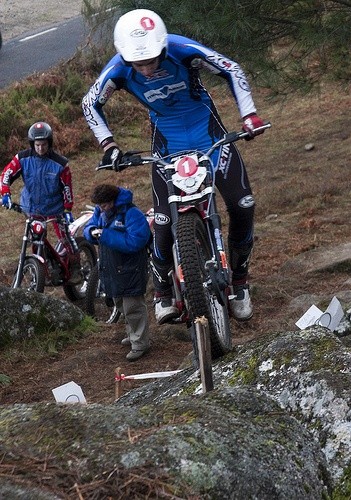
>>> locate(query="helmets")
[113,9,168,66]
[28,122,53,149]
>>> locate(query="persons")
[0,122,90,293]
[83,9,263,322]
[83,184,152,360]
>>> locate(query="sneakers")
[227,280,254,321]
[152,297,179,325]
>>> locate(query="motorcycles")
[80,204,158,325]
[95,123,275,361]
[1,199,97,302]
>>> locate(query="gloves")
[2,195,12,209]
[241,116,264,140]
[63,212,73,224]
[102,146,123,173]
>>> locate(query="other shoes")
[126,350,144,359]
[121,337,131,346]
[68,270,82,282]
